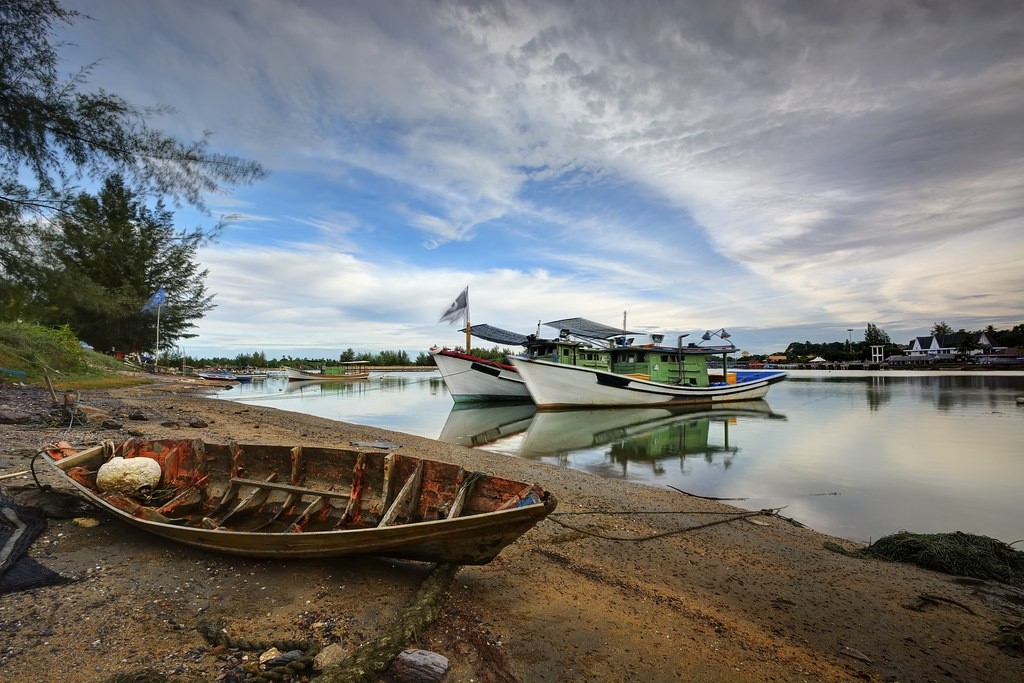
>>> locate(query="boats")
[503,308,792,412]
[515,397,788,477]
[195,371,270,381]
[37,435,559,567]
[281,365,370,381]
[439,400,540,448]
[428,324,611,404]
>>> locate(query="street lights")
[846,329,853,352]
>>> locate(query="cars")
[763,365,774,369]
[79,341,95,350]
[136,354,151,364]
